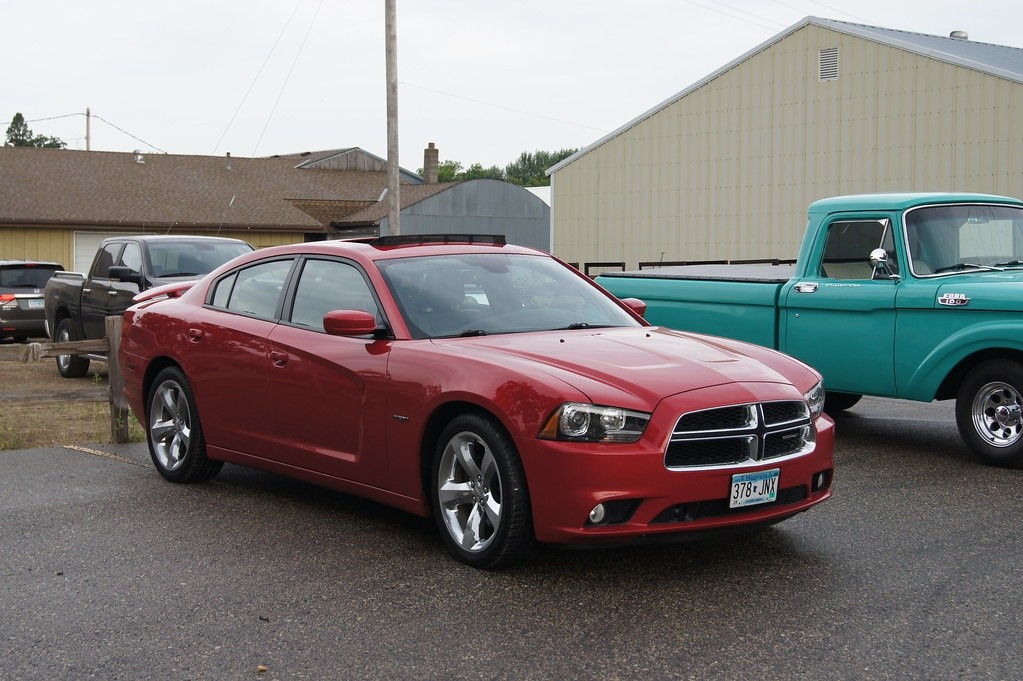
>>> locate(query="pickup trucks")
[590,192,1023,470]
[42,234,259,379]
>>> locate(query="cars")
[0,259,66,344]
[118,230,834,570]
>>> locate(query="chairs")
[410,266,485,340]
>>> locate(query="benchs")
[817,260,934,281]
